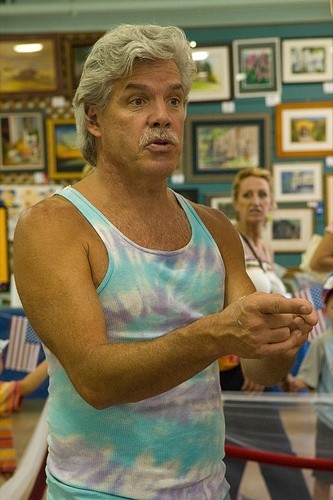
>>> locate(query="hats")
[321,273,333,304]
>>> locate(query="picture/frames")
[276,101,333,158]
[231,36,282,99]
[184,114,269,183]
[0,31,106,180]
[188,43,233,105]
[282,36,333,85]
[204,162,333,254]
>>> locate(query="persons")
[13,24,318,500]
[223,168,312,500]
[308,222,333,273]
[0,342,47,482]
[278,289,333,500]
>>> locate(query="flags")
[6,316,40,374]
[300,285,325,340]
